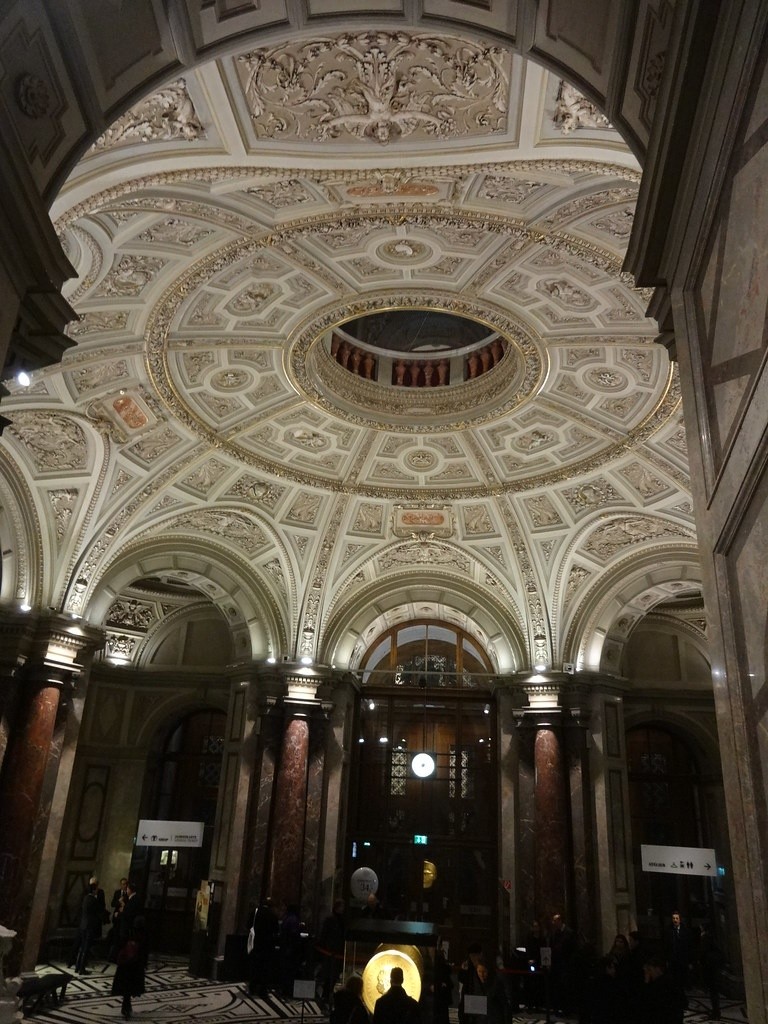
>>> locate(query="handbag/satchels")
[114,928,139,968]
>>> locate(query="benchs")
[17,973,73,1018]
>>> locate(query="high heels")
[121,1000,133,1021]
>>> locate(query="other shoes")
[75,969,92,975]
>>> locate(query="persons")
[332,975,370,1024]
[455,945,512,1024]
[110,903,151,1022]
[67,876,111,975]
[106,878,141,965]
[373,968,423,1024]
[665,912,722,1021]
[525,914,581,1014]
[425,944,454,1024]
[588,930,687,1024]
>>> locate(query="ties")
[675,925,678,936]
[122,891,125,896]
